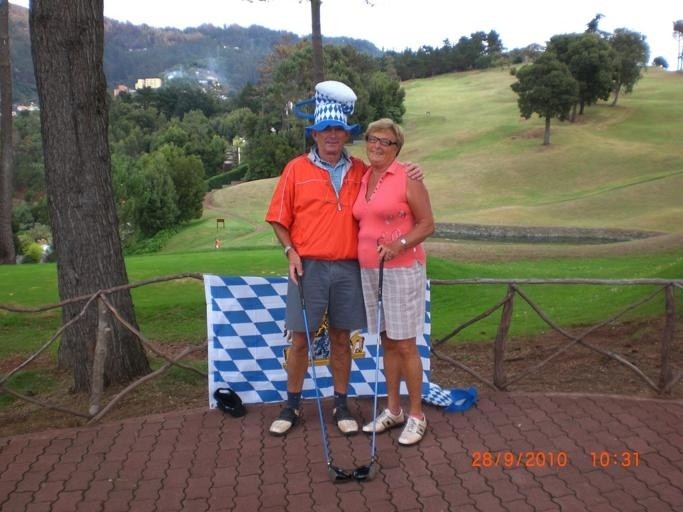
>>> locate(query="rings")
[388,255,392,259]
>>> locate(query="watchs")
[396,236,410,252]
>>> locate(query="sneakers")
[362,408,404,432]
[269,406,303,436]
[398,414,427,445]
[333,405,359,435]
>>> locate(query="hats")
[213,387,246,416]
[304,103,360,139]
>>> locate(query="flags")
[201,272,432,411]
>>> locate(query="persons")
[265,80,424,437]
[349,118,436,446]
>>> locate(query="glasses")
[365,134,398,146]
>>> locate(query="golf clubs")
[297,258,351,483]
[352,254,383,481]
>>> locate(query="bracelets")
[283,245,294,256]
